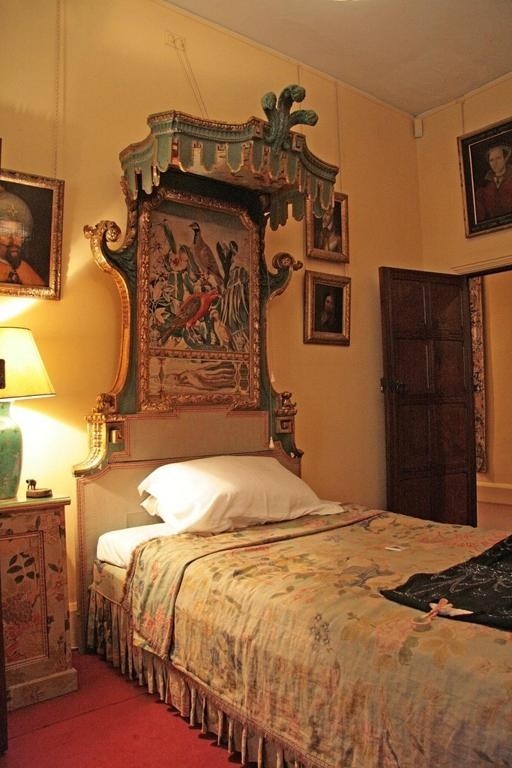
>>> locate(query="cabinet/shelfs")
[1,168,65,302]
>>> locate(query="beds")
[73,82,512,768]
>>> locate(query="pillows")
[136,455,345,536]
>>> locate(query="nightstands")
[0,496,79,713]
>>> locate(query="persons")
[0,191,47,286]
[475,144,512,222]
[315,209,343,253]
[315,294,341,331]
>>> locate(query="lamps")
[0,326,57,499]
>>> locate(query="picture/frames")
[456,117,512,239]
[304,190,349,264]
[303,268,352,347]
